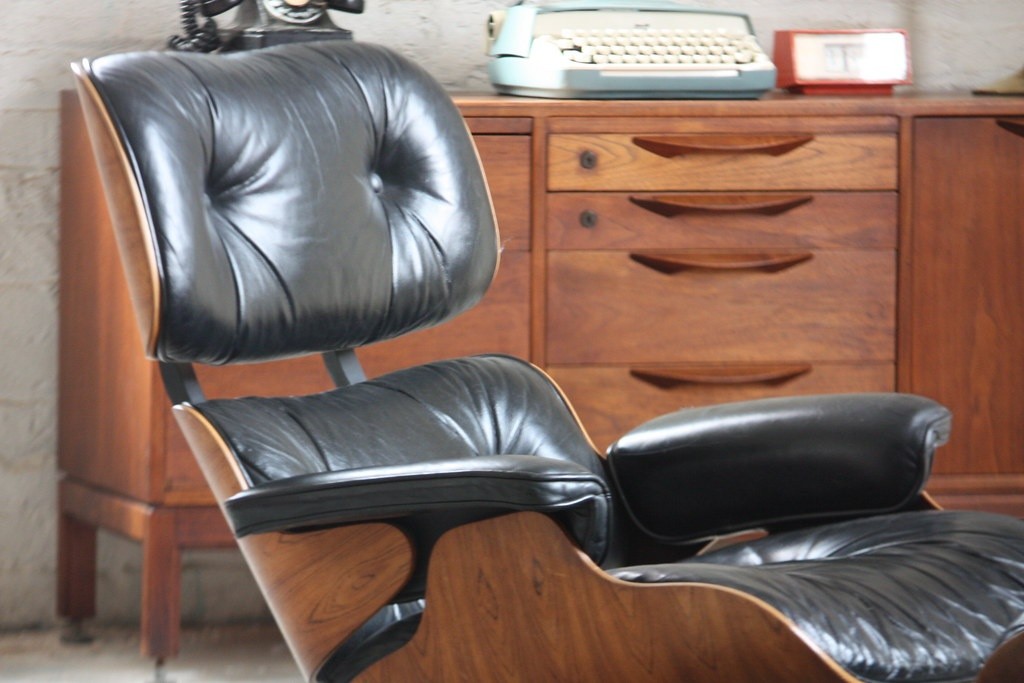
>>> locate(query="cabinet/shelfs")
[53,84,535,658]
[898,98,1023,523]
[537,100,899,456]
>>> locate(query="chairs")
[70,40,1024,682]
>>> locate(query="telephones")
[166,0,365,57]
